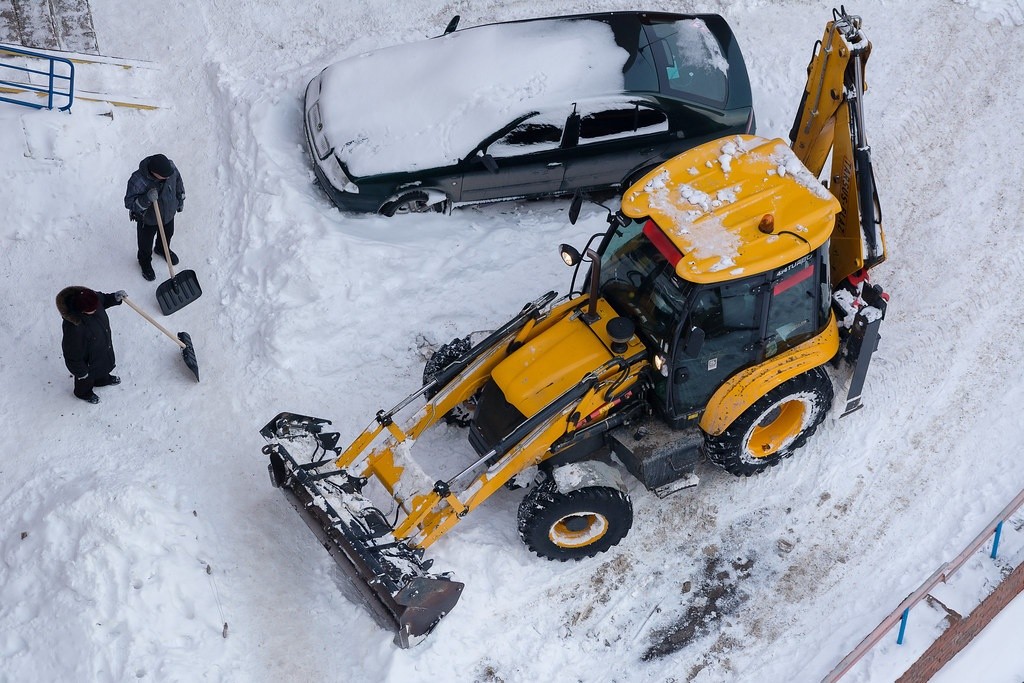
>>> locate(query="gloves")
[78,373,88,380]
[148,188,158,201]
[115,290,128,301]
[177,205,184,212]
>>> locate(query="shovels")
[122,296,199,382]
[154,199,202,316]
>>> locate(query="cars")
[303,12,759,215]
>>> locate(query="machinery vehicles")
[258,9,891,647]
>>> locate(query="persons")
[124,153,186,282]
[55,286,129,404]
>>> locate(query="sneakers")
[138,260,156,281]
[154,247,179,265]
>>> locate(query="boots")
[90,372,120,387]
[73,377,100,404]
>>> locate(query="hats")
[77,290,99,312]
[149,154,174,177]
